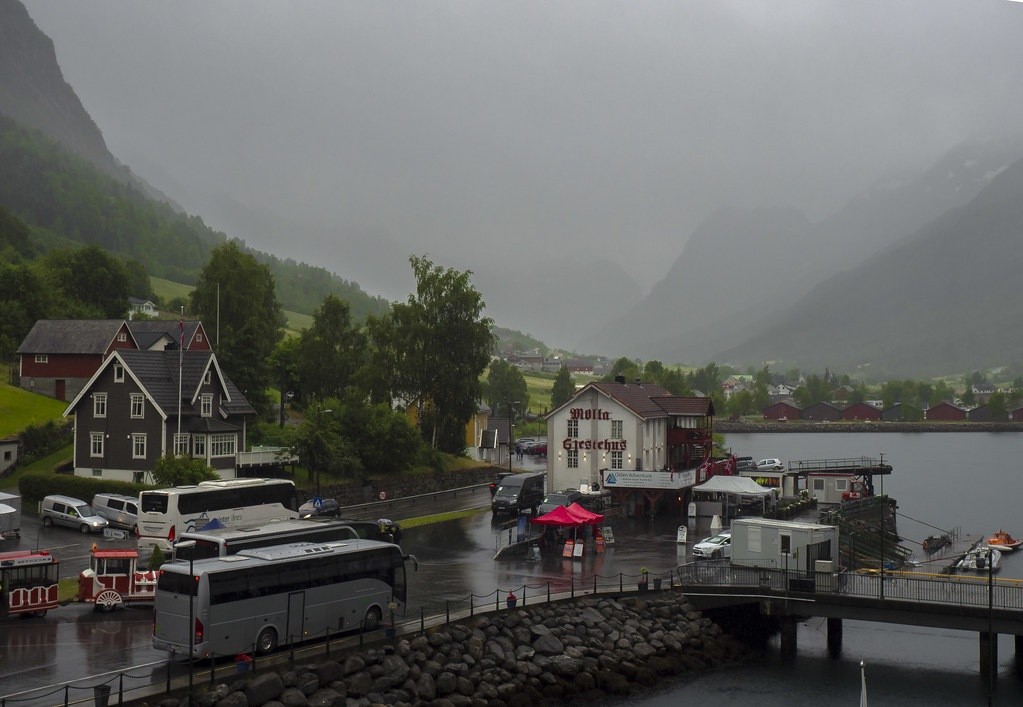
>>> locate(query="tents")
[529,504,592,543]
[691,475,778,516]
[568,502,604,527]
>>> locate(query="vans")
[37,495,109,534]
[92,493,139,536]
[491,474,545,517]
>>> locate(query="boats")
[956,527,1023,573]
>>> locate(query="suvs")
[735,456,784,472]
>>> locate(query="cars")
[693,533,731,562]
[299,499,342,519]
[539,489,583,516]
[514,438,547,457]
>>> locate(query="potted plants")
[765,490,818,519]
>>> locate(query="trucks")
[0,492,22,540]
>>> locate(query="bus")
[0,543,160,620]
[138,478,301,552]
[151,518,419,660]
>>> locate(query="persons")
[515,443,524,460]
[886,560,895,577]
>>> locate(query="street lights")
[509,401,520,472]
[315,406,333,427]
[878,452,887,599]
[537,401,541,441]
